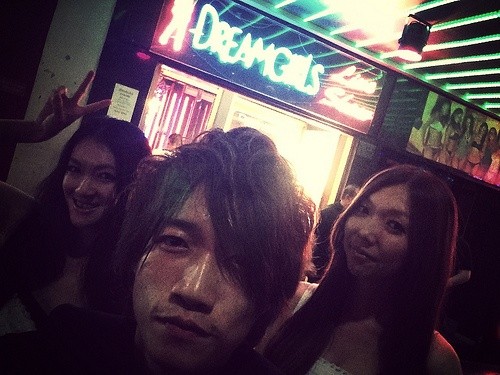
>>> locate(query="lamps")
[396,13,432,63]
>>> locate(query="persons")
[419,97,500,186]
[0,71,463,375]
